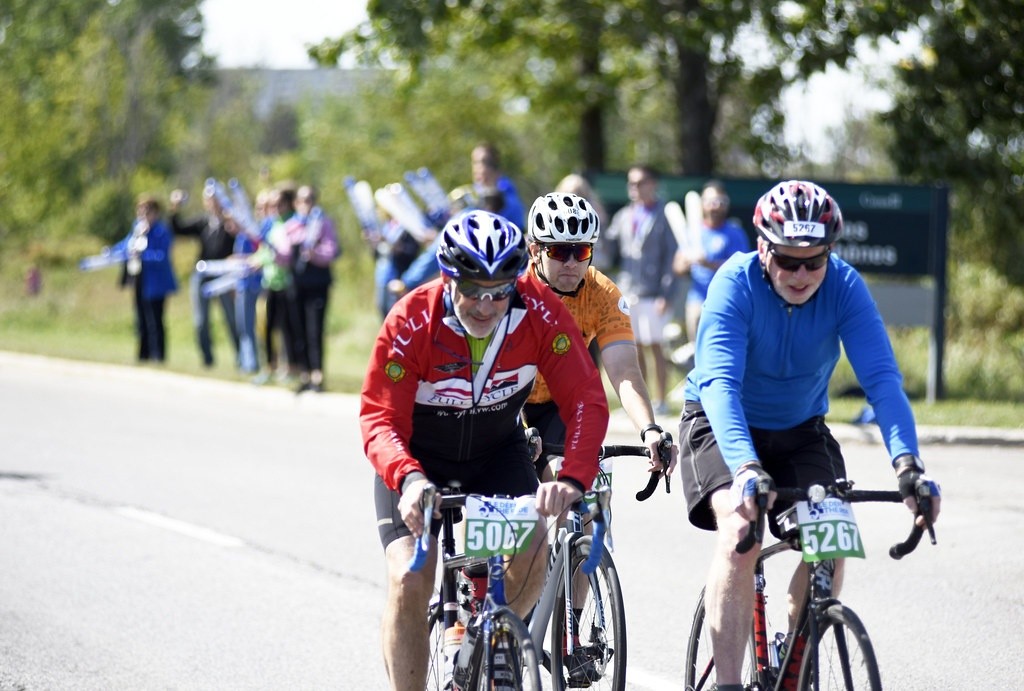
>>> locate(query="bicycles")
[525,425,671,691]
[407,480,615,691]
[684,475,937,691]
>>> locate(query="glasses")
[766,243,833,273]
[451,277,516,301]
[536,242,595,262]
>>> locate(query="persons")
[369,140,526,320]
[556,173,612,276]
[166,185,338,393]
[363,209,609,691]
[114,197,178,362]
[608,164,678,413]
[443,192,679,691]
[678,178,941,691]
[672,183,749,360]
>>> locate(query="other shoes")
[561,646,596,688]
[777,643,789,670]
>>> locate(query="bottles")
[457,578,478,626]
[442,615,485,691]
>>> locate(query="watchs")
[641,424,664,442]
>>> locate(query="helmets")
[435,210,529,280]
[528,192,601,244]
[752,180,845,249]
